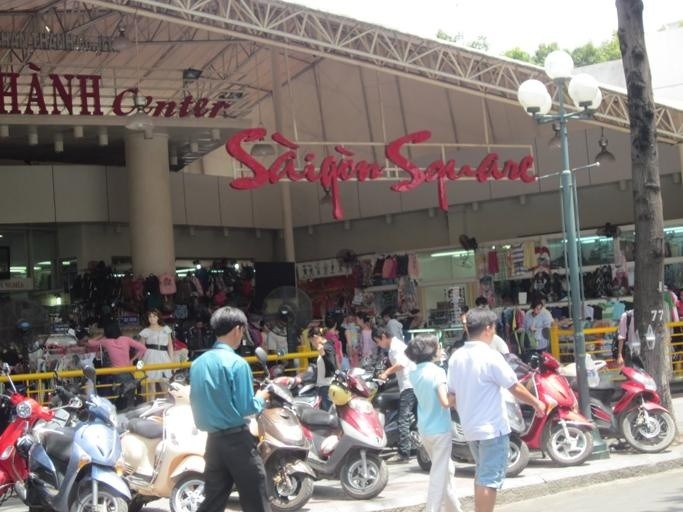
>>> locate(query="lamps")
[593,127,616,163]
[548,121,562,149]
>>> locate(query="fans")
[596,223,621,238]
[0,299,51,375]
[262,285,313,370]
[336,247,357,268]
[459,234,478,250]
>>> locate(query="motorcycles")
[366,347,677,479]
[0,348,390,512]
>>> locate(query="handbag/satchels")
[611,338,634,364]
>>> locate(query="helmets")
[15,432,33,457]
[329,380,355,406]
[320,435,338,459]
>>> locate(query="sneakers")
[385,454,410,464]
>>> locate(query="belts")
[211,425,249,440]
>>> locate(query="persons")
[188,306,272,512]
[403,334,465,512]
[446,306,547,512]
[80,319,147,397]
[137,307,174,404]
[370,326,420,466]
[663,285,683,372]
[249,306,404,412]
[223,264,255,312]
[475,295,554,364]
[459,312,509,361]
[617,309,644,376]
